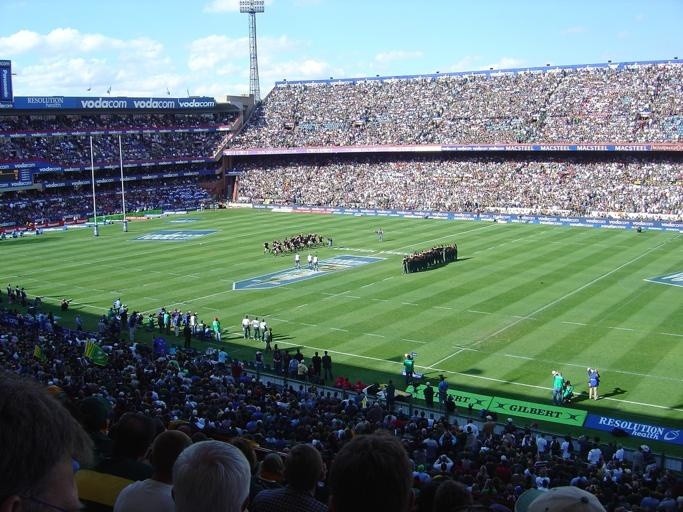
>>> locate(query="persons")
[0,64,683,511]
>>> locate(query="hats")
[515,486,606,512]
